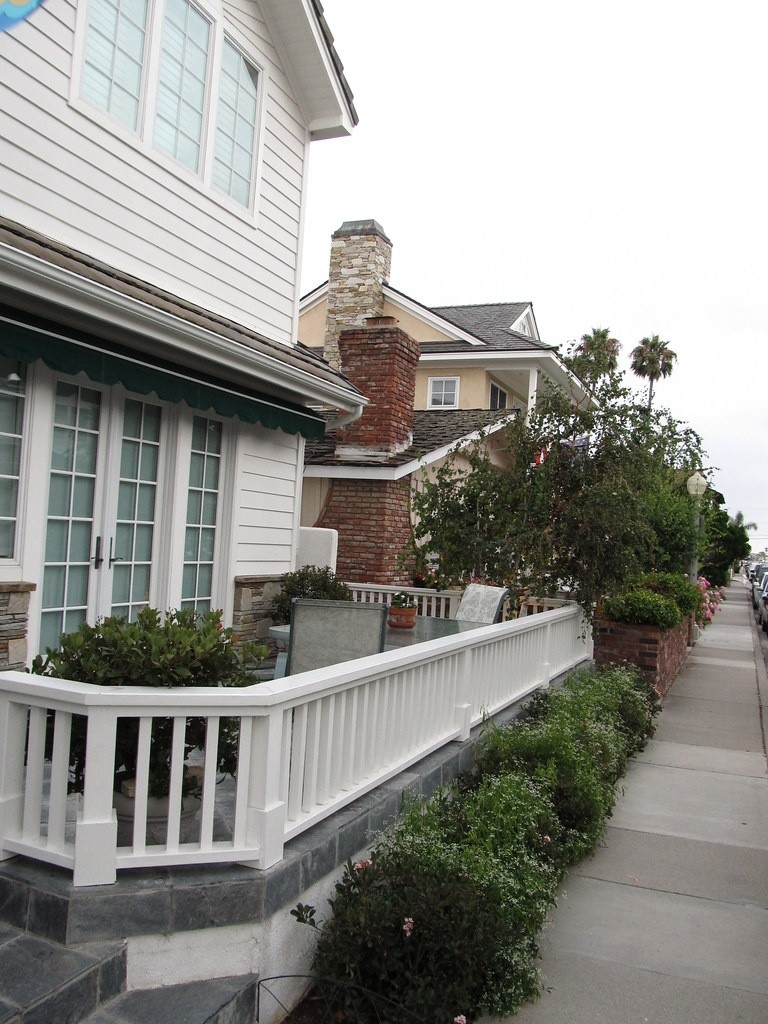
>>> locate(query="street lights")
[686,471,707,647]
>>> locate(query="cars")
[741,558,768,637]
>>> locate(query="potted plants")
[20,604,270,848]
[269,564,354,652]
[387,590,418,629]
